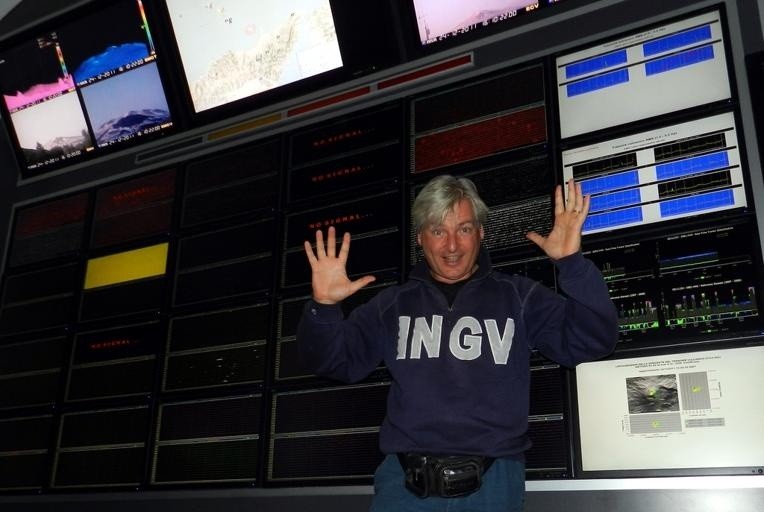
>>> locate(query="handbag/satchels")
[398,452,492,499]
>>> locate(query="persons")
[294,174,620,512]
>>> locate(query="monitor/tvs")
[580,215,763,352]
[550,3,739,146]
[552,103,756,246]
[566,338,764,479]
